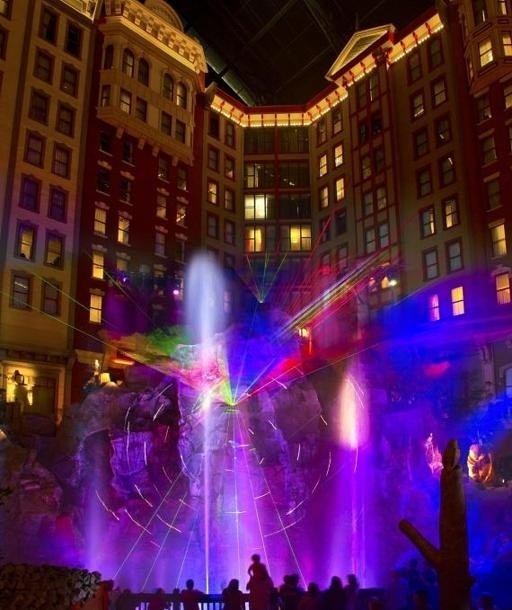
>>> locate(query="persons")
[2,554,495,609]
